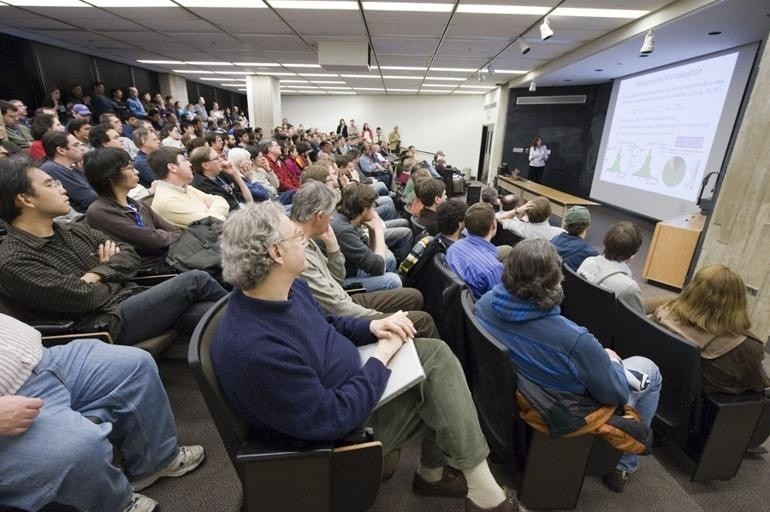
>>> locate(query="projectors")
[318,41,373,74]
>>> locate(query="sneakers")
[123,493,160,512]
[465,489,530,512]
[132,445,205,492]
[601,468,636,492]
[414,464,468,497]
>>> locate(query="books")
[356,335,426,415]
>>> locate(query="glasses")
[282,231,304,241]
[48,180,62,193]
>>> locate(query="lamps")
[528,78,539,92]
[517,17,558,57]
[638,31,658,54]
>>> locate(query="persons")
[646,265,770,460]
[211,198,523,512]
[0,82,657,348]
[0,310,205,512]
[471,238,664,492]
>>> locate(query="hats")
[73,103,92,115]
[564,206,591,226]
